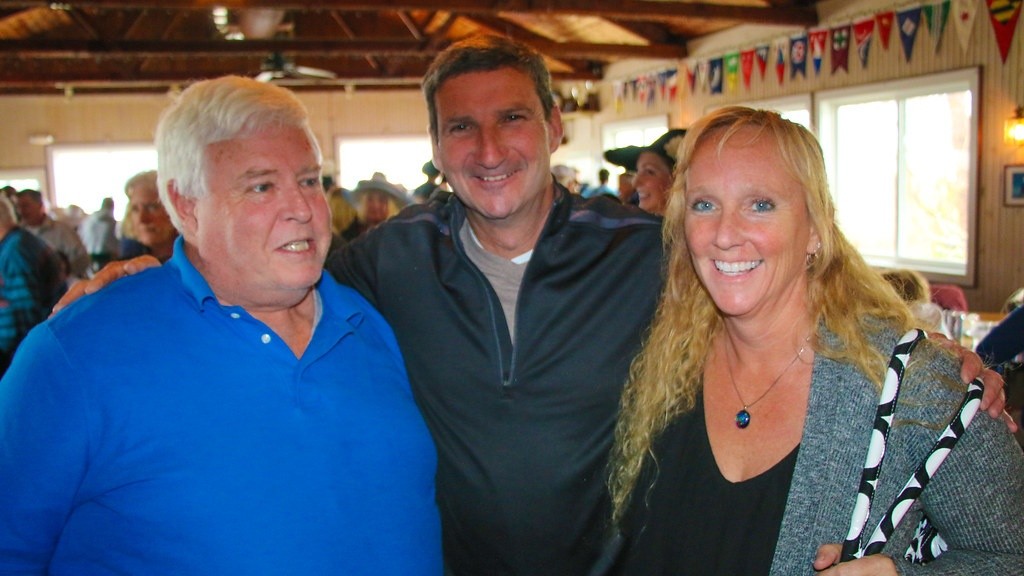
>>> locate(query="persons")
[47,33,1017,576]
[600,107,1024,576]
[0,75,443,575]
[882,269,1024,370]
[0,129,692,379]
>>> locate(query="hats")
[603,129,687,170]
[357,172,405,206]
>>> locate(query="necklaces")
[723,331,810,430]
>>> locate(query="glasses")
[127,201,164,215]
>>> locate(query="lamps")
[1004,104,1024,145]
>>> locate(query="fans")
[254,52,336,82]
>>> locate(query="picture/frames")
[1003,164,1024,207]
